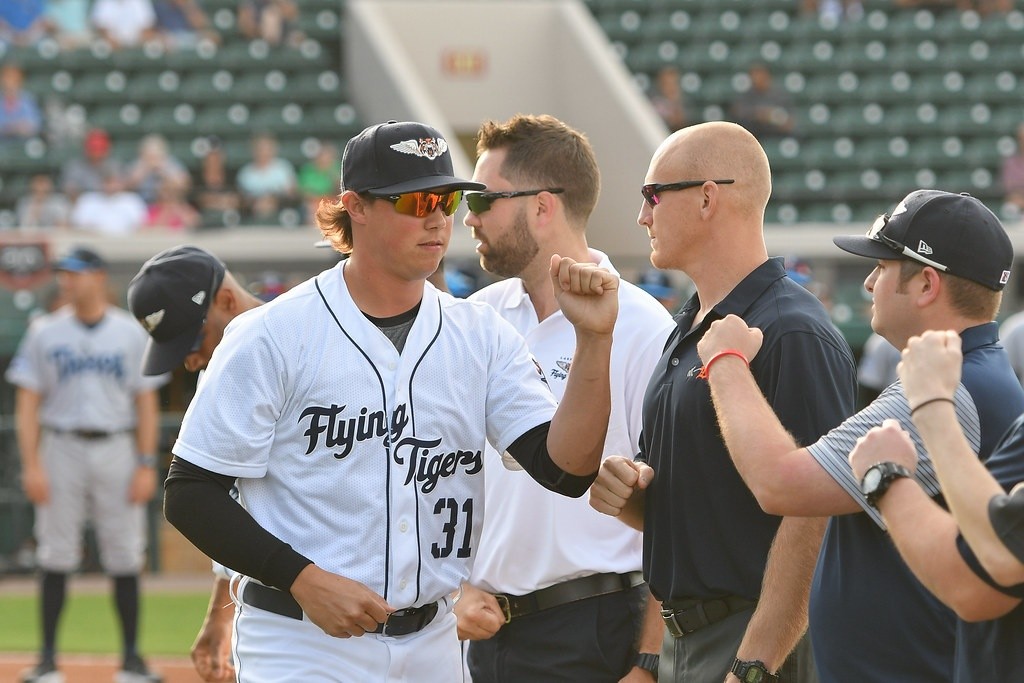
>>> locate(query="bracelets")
[139,455,159,465]
[909,398,956,416]
[634,652,659,683]
[704,349,749,379]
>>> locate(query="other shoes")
[116,663,160,683]
[23,665,57,683]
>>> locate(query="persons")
[697,189,1024,683]
[17,128,342,233]
[0,63,42,138]
[3,250,171,683]
[652,62,796,134]
[463,115,678,683]
[0,0,308,51]
[164,121,619,683]
[637,121,859,683]
[634,269,677,312]
[896,330,1024,587]
[128,244,265,683]
[848,413,1024,683]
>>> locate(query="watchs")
[860,462,912,511]
[731,657,779,683]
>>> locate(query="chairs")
[581,0,1024,232]
[0,0,373,230]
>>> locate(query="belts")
[494,567,645,624]
[660,586,767,640]
[76,429,107,439]
[244,583,448,638]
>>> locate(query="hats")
[342,119,487,196]
[86,129,112,157]
[125,245,227,376]
[55,247,106,272]
[833,188,1014,290]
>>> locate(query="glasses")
[865,212,948,272]
[465,187,567,217]
[361,188,463,218]
[641,178,735,208]
